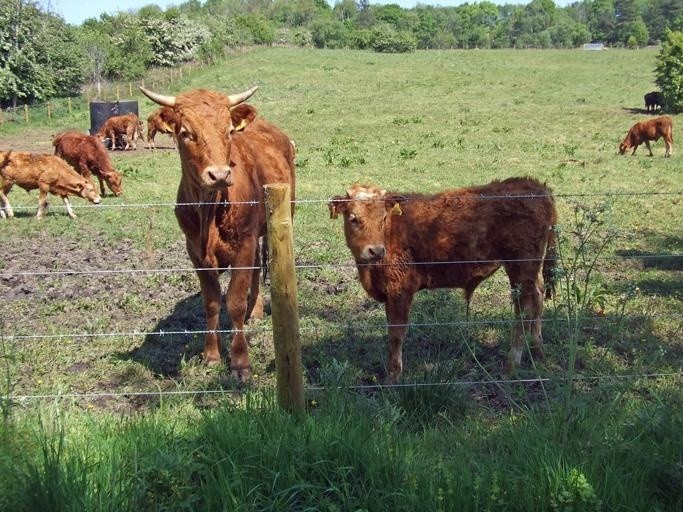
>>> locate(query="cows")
[329,177,558,385]
[619,117,673,158]
[147,109,173,152]
[95,113,145,151]
[137,85,297,384]
[0,151,103,221]
[644,91,661,112]
[53,131,123,198]
